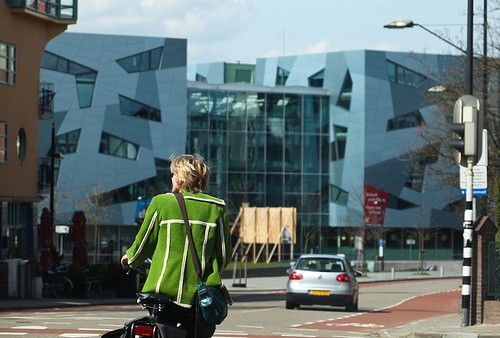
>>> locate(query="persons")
[121,154,233,338]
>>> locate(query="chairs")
[309,263,316,270]
[332,264,341,271]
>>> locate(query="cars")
[286,253,359,312]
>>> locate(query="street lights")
[384,17,486,131]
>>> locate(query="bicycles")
[121,256,172,338]
[42,268,73,298]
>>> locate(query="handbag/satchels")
[195,281,233,325]
